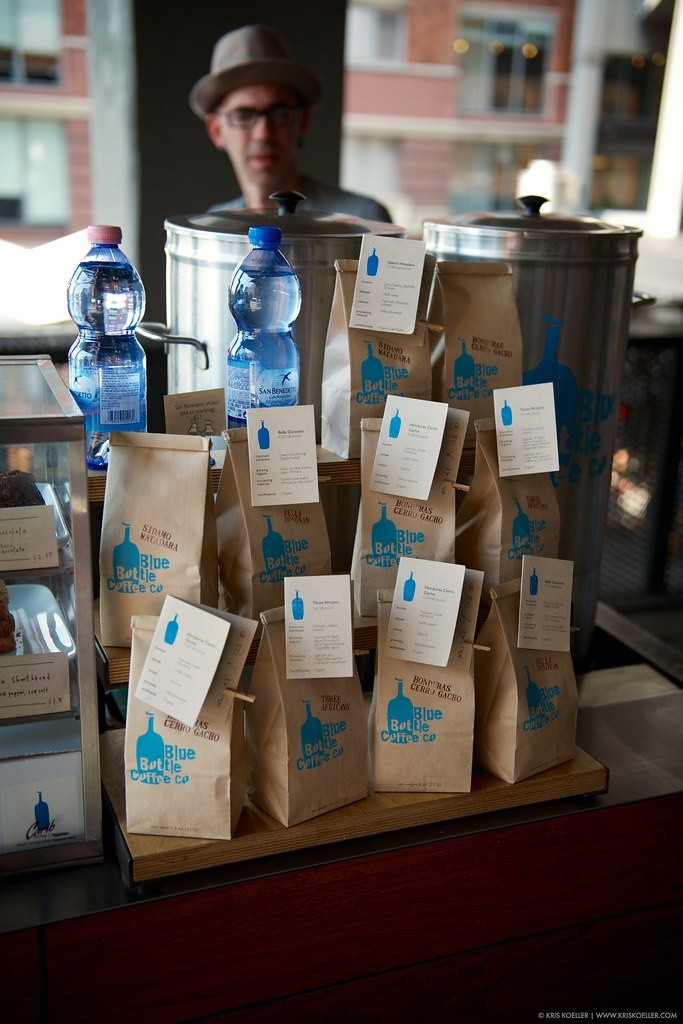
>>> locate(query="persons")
[189,25,391,223]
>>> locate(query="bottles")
[67,223,149,470]
[227,227,302,427]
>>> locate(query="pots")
[164,190,410,398]
[422,196,657,664]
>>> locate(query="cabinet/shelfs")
[0,353,104,880]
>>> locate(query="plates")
[7,583,77,662]
[35,482,70,550]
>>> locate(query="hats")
[190,25,324,121]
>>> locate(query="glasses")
[217,105,302,127]
